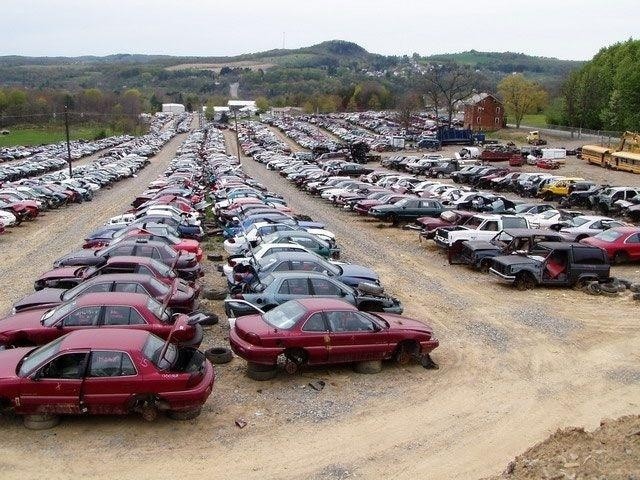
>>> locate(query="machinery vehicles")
[526,131,547,146]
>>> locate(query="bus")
[580,146,610,167]
[610,152,640,172]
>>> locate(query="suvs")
[489,242,610,290]
[433,215,531,251]
[461,228,565,270]
[450,164,586,200]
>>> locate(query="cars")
[0,164,90,234]
[227,233,381,290]
[262,153,448,223]
[528,209,585,232]
[0,278,203,350]
[230,109,464,159]
[34,251,200,313]
[580,226,640,265]
[132,171,287,207]
[246,224,332,260]
[0,314,214,415]
[89,165,131,199]
[107,206,204,239]
[223,217,340,256]
[83,227,203,262]
[12,274,200,314]
[134,135,240,170]
[143,110,228,134]
[557,207,634,242]
[223,240,345,290]
[84,215,200,242]
[0,135,132,164]
[223,264,402,319]
[229,298,438,367]
[53,238,204,281]
[222,214,325,238]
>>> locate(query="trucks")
[430,161,471,179]
[527,148,566,166]
